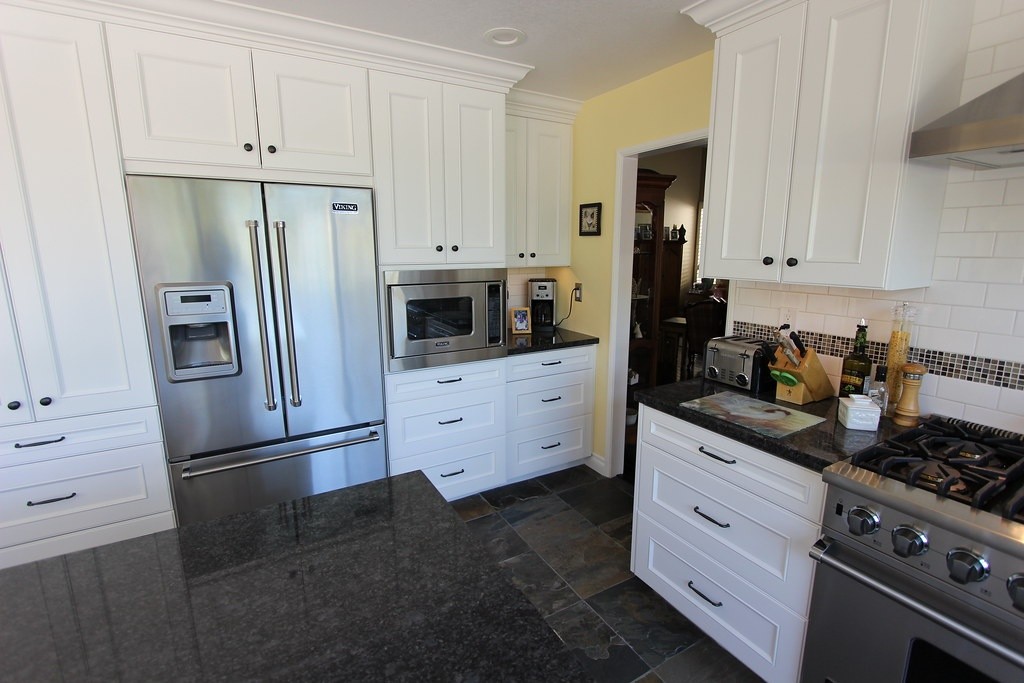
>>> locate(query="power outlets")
[779,306,799,335]
[575,282,582,302]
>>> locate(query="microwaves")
[381,266,508,374]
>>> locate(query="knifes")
[762,327,807,369]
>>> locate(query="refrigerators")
[127,173,388,529]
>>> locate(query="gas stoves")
[821,415,1023,626]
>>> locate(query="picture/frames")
[579,202,602,237]
[510,307,532,334]
[638,224,651,234]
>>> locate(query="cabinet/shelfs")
[629,168,687,385]
[0,0,599,570]
[680,0,973,291]
[629,403,828,683]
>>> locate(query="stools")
[661,317,687,368]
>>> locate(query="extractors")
[908,72,1024,169]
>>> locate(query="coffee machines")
[527,279,557,330]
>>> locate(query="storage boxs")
[838,397,881,432]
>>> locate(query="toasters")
[705,334,780,393]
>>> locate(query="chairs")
[684,300,718,365]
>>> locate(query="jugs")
[533,303,552,325]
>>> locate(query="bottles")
[671,224,678,240]
[838,319,873,405]
[883,301,914,409]
[678,224,686,240]
[869,365,890,416]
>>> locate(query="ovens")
[798,533,1023,683]
